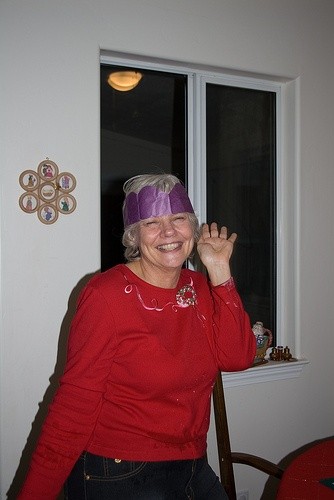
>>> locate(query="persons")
[18,175,256,500]
